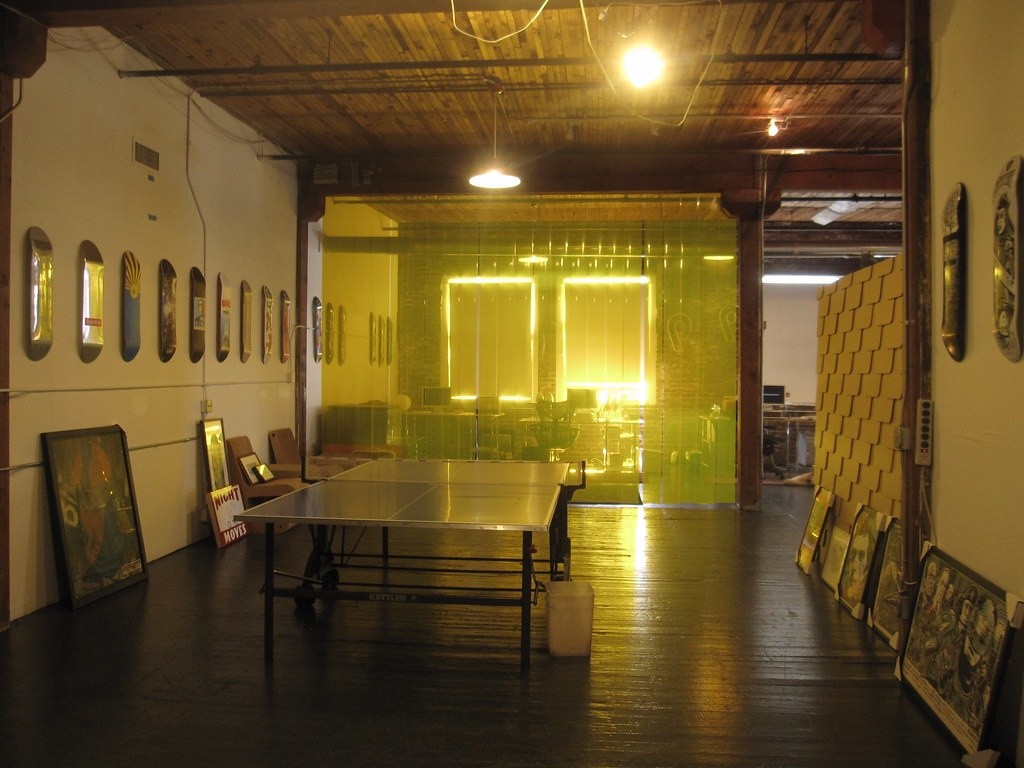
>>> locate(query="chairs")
[469,394,499,461]
[225,435,311,534]
[269,428,345,478]
[530,401,581,462]
[763,426,785,480]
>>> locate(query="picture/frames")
[833,501,887,620]
[200,416,231,492]
[795,485,837,576]
[252,463,276,483]
[865,514,930,651]
[892,540,1024,768]
[41,425,149,611]
[237,452,262,487]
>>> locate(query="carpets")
[568,484,643,505]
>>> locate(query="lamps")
[469,93,521,188]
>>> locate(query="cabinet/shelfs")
[697,413,733,471]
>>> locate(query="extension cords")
[915,399,933,464]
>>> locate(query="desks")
[517,417,645,474]
[267,463,322,481]
[232,481,562,669]
[405,410,506,460]
[315,456,570,595]
